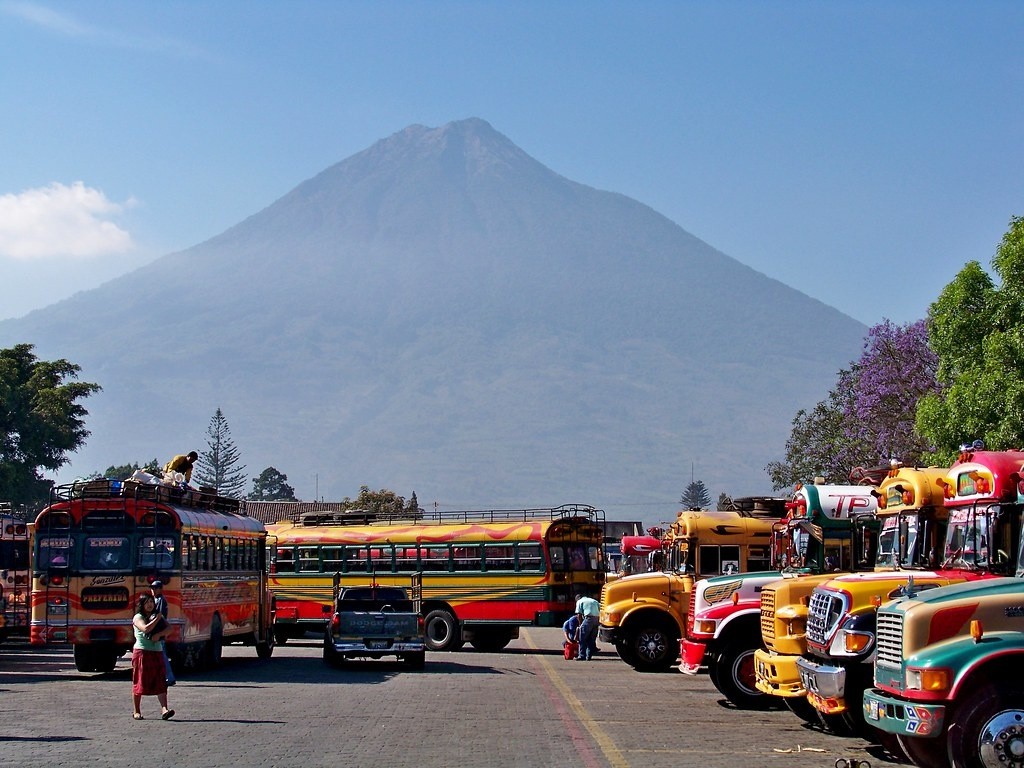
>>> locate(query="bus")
[257,502,608,655]
[34,477,274,674]
[0,503,37,639]
[598,439,1024,768]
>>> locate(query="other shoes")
[161,709,175,720]
[132,712,144,720]
[575,657,591,661]
[165,680,176,686]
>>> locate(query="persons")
[562,593,602,662]
[145,580,169,643]
[130,593,173,719]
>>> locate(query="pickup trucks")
[320,568,426,670]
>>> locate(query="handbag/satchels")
[143,614,167,641]
[565,642,579,661]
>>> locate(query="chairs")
[140,544,176,569]
[276,550,544,573]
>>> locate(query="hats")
[569,617,580,630]
[148,581,163,590]
